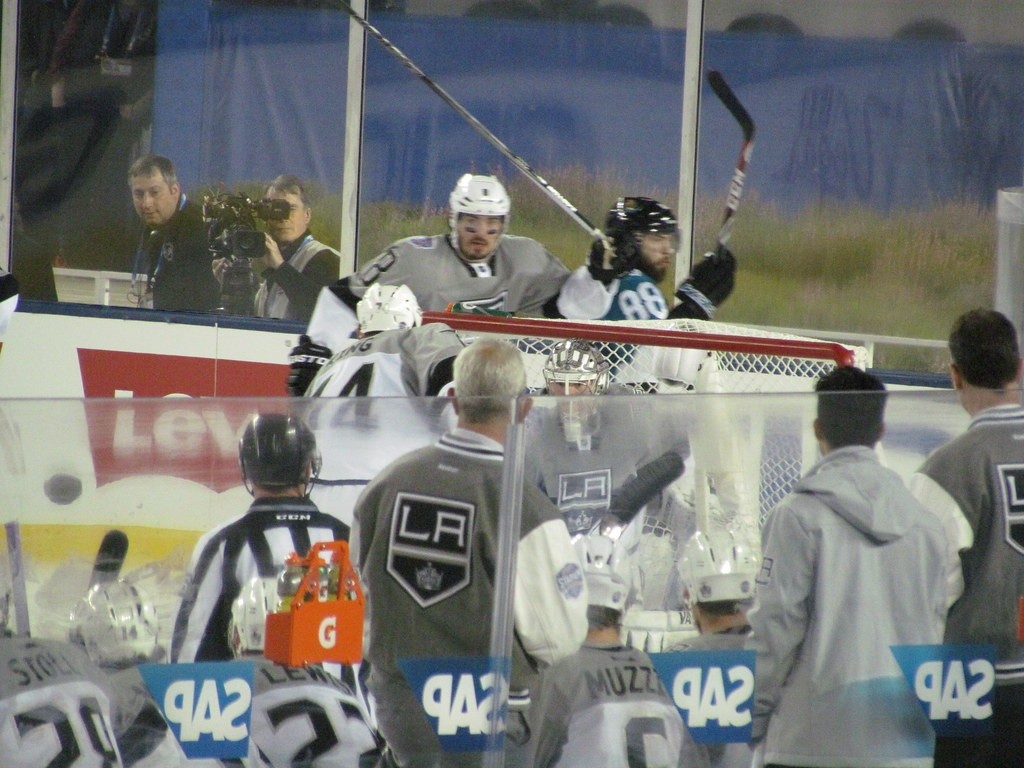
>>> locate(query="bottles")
[276,554,356,614]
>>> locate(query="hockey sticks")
[705,66,758,258]
[601,451,685,545]
[347,4,602,240]
[4,520,30,639]
[87,527,130,611]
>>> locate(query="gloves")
[672,244,737,321]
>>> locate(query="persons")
[130,155,220,311]
[212,180,339,323]
[287,174,639,424]
[15,1,153,303]
[521,195,754,768]
[350,339,589,768]
[917,311,1024,768]
[751,366,947,768]
[306,281,461,528]
[0,412,390,768]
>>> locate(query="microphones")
[219,206,235,226]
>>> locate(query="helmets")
[542,338,611,398]
[634,520,764,609]
[446,172,513,265]
[69,580,160,669]
[354,282,425,333]
[604,195,685,283]
[228,577,277,654]
[570,531,636,614]
[237,413,325,502]
